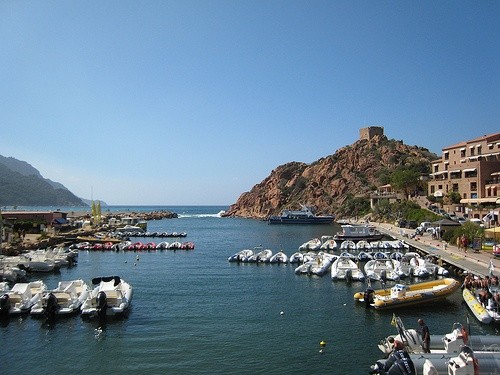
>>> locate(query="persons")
[493,292,500,314]
[388,337,404,352]
[432,228,437,239]
[461,234,465,250]
[447,207,450,213]
[415,318,431,353]
[478,290,492,308]
[462,238,468,253]
[463,272,499,289]
[456,236,461,251]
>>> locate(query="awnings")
[55,218,67,224]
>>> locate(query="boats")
[268,209,335,224]
[29,280,89,316]
[333,224,385,242]
[0,248,78,280]
[0,280,48,315]
[228,249,449,281]
[299,238,408,250]
[369,328,500,375]
[80,276,132,317]
[108,230,187,238]
[61,241,194,249]
[354,278,460,311]
[463,285,500,323]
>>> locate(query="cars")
[397,219,435,233]
[428,205,484,228]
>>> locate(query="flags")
[483,213,494,222]
